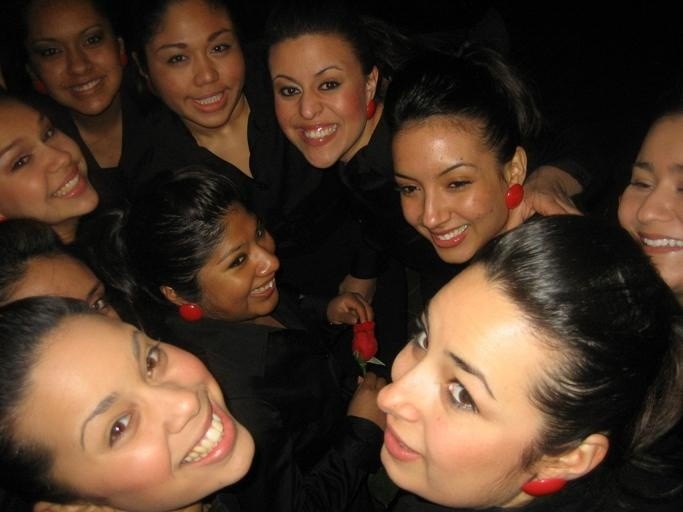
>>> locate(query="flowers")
[351,322,386,377]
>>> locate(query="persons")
[2,0,683,511]
